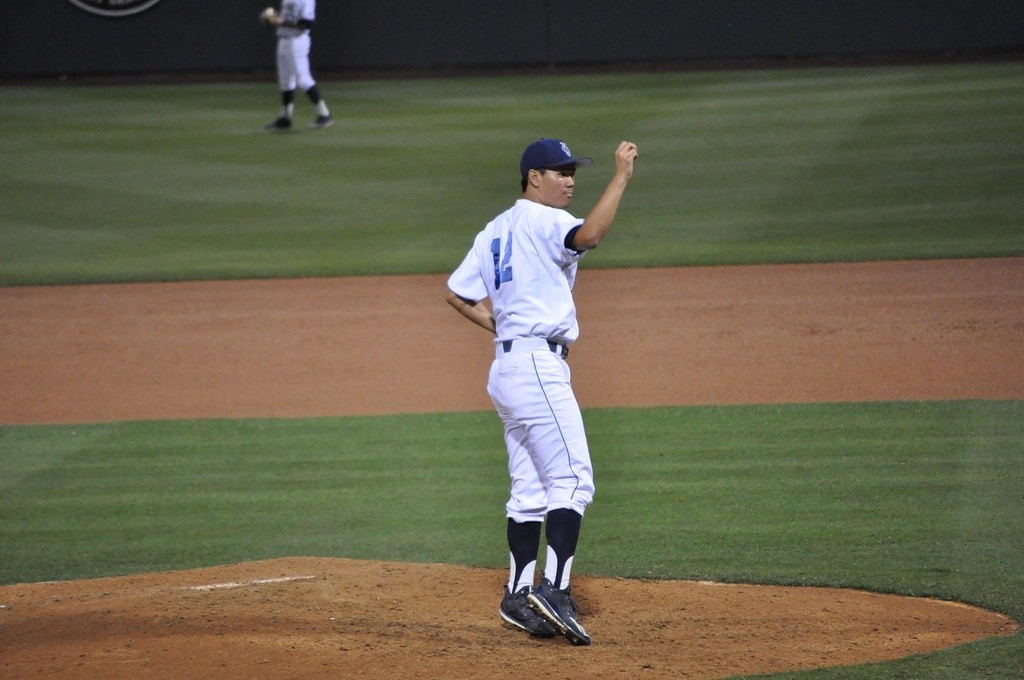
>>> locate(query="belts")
[502,339,569,358]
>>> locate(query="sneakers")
[500,585,557,636]
[526,577,591,645]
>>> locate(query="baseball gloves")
[259,6,280,28]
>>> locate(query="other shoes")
[308,115,334,128]
[264,117,292,130]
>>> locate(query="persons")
[259,0,335,132]
[446,139,638,647]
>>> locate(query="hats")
[519,138,593,173]
